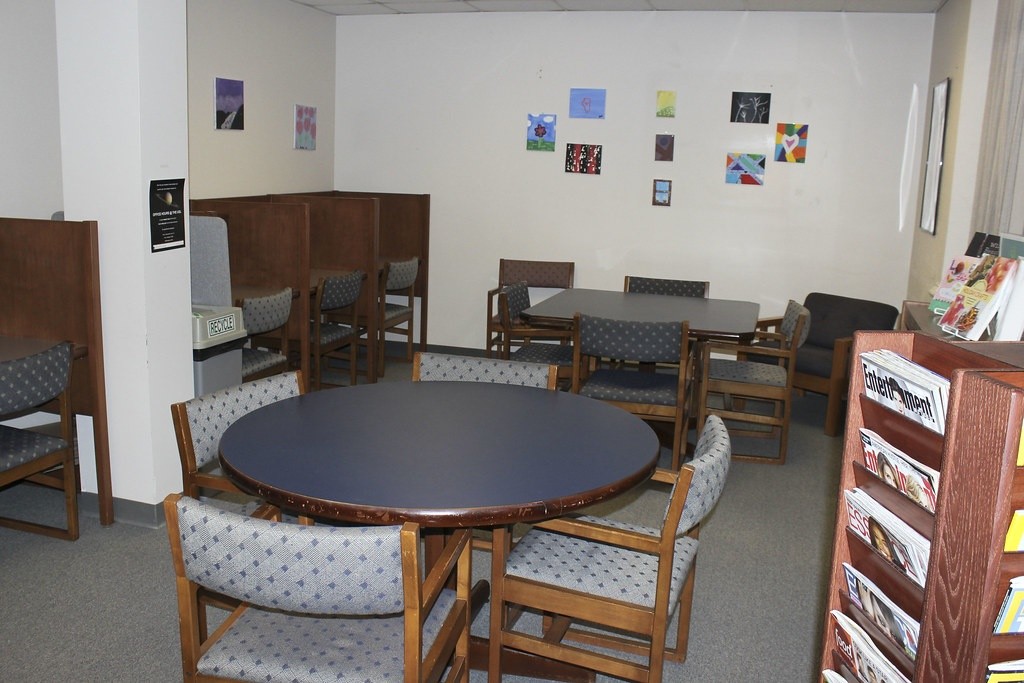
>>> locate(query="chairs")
[325,256,419,377]
[487,259,575,391]
[572,314,697,474]
[738,293,900,437]
[624,276,711,299]
[235,286,293,382]
[697,299,812,465]
[164,351,732,683]
[0,340,79,541]
[252,268,362,390]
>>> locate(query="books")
[922,250,1019,340]
[983,417,1024,683]
[818,348,951,683]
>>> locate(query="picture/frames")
[919,77,949,236]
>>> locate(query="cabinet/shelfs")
[818,329,1024,683]
[899,301,998,342]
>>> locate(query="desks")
[520,289,761,459]
[219,381,660,683]
[0,217,114,526]
[189,190,430,393]
